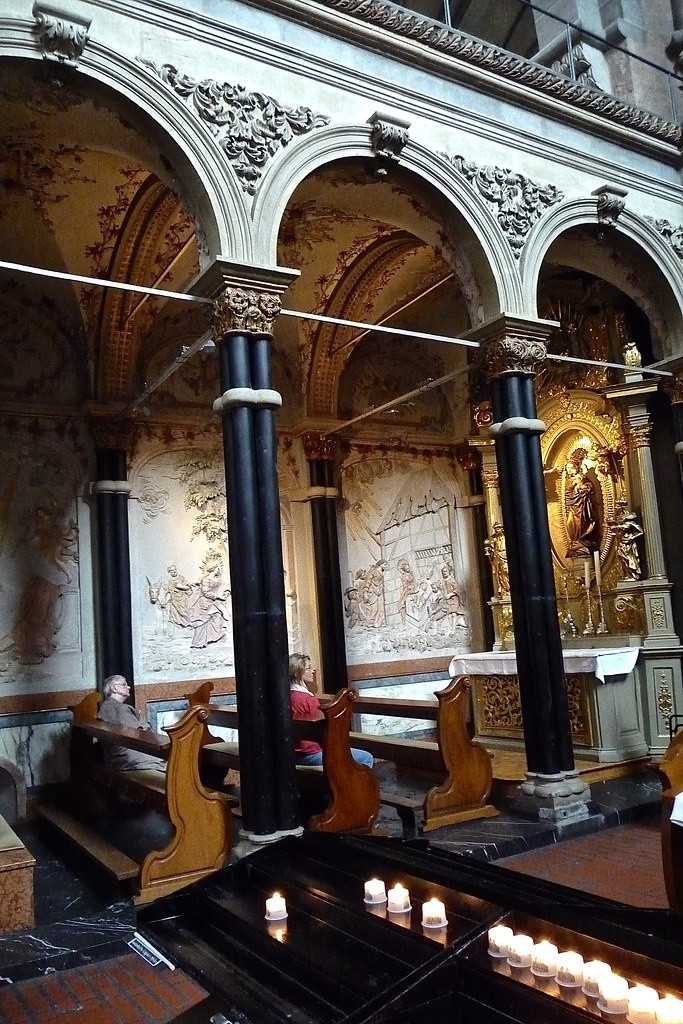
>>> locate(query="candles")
[594,550,603,586]
[267,920,287,943]
[595,974,629,1016]
[555,950,584,988]
[582,960,610,996]
[584,562,591,588]
[505,933,534,968]
[656,993,683,1024]
[266,890,290,920]
[529,938,559,978]
[420,896,449,929]
[626,984,659,1024]
[488,923,514,958]
[362,877,388,904]
[386,881,413,914]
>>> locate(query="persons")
[290,651,373,772]
[97,675,236,796]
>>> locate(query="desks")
[293,670,501,831]
[449,648,648,765]
[102,680,379,838]
[28,691,244,904]
